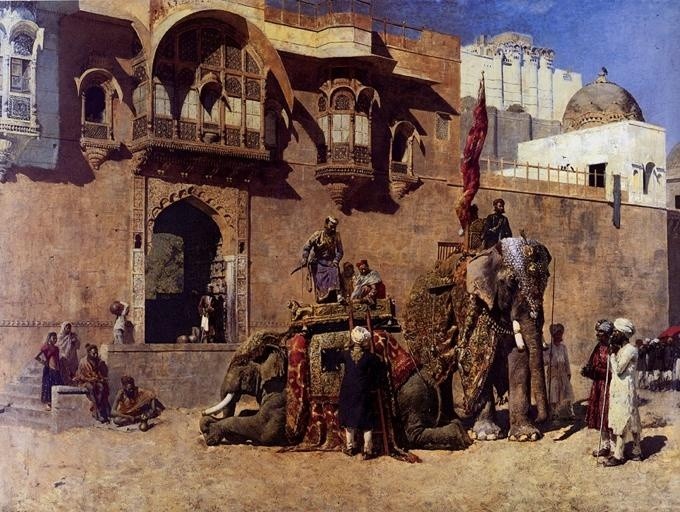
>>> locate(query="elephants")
[198,330,476,451]
[402,234,557,441]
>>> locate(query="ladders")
[348,309,390,457]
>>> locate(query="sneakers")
[629,451,646,462]
[362,446,379,461]
[343,446,354,456]
[604,455,628,468]
[588,446,610,457]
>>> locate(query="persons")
[109,374,162,427]
[74,341,111,423]
[112,300,130,346]
[633,334,680,392]
[331,324,384,461]
[540,321,575,427]
[339,258,357,296]
[479,197,512,251]
[34,330,64,412]
[302,215,346,303]
[56,321,81,383]
[602,315,647,467]
[351,259,387,306]
[197,283,214,343]
[580,316,616,458]
[210,286,228,343]
